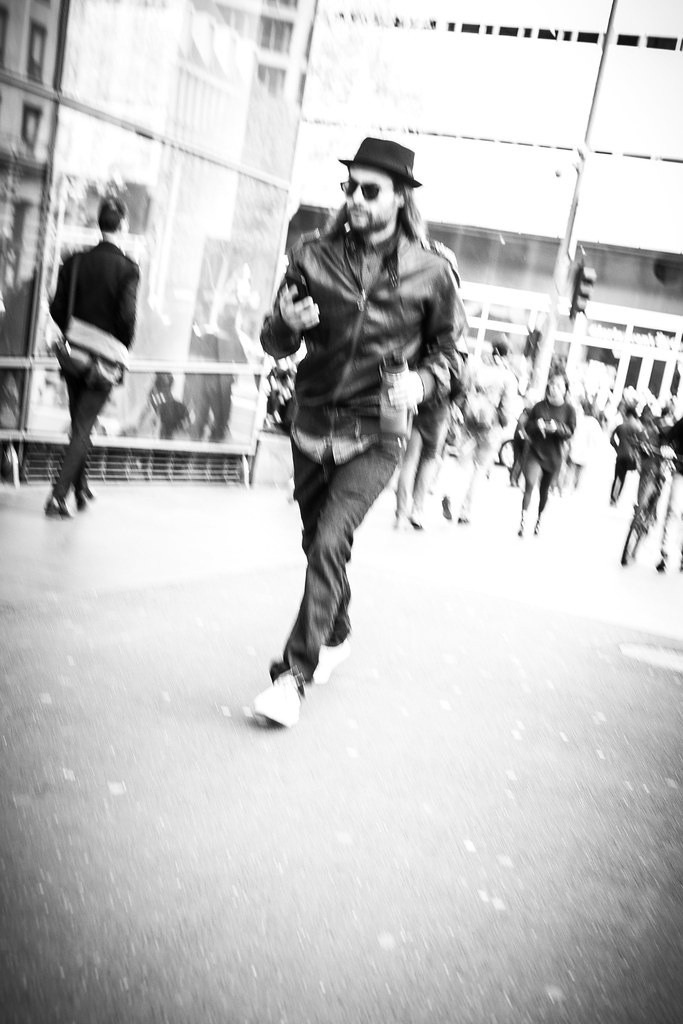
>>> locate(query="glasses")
[338,179,400,201]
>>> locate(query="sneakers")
[311,644,355,682]
[253,677,301,731]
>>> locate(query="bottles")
[378,348,408,453]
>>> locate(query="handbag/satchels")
[49,316,128,388]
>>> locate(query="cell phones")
[285,273,309,304]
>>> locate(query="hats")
[337,136,422,186]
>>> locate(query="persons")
[252,137,469,724]
[41,195,141,520]
[183,281,244,445]
[387,334,683,579]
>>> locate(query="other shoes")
[517,508,543,536]
[46,495,71,519]
[656,554,671,572]
[441,496,471,524]
[397,514,423,531]
[75,491,94,511]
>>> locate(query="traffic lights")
[569,266,599,322]
